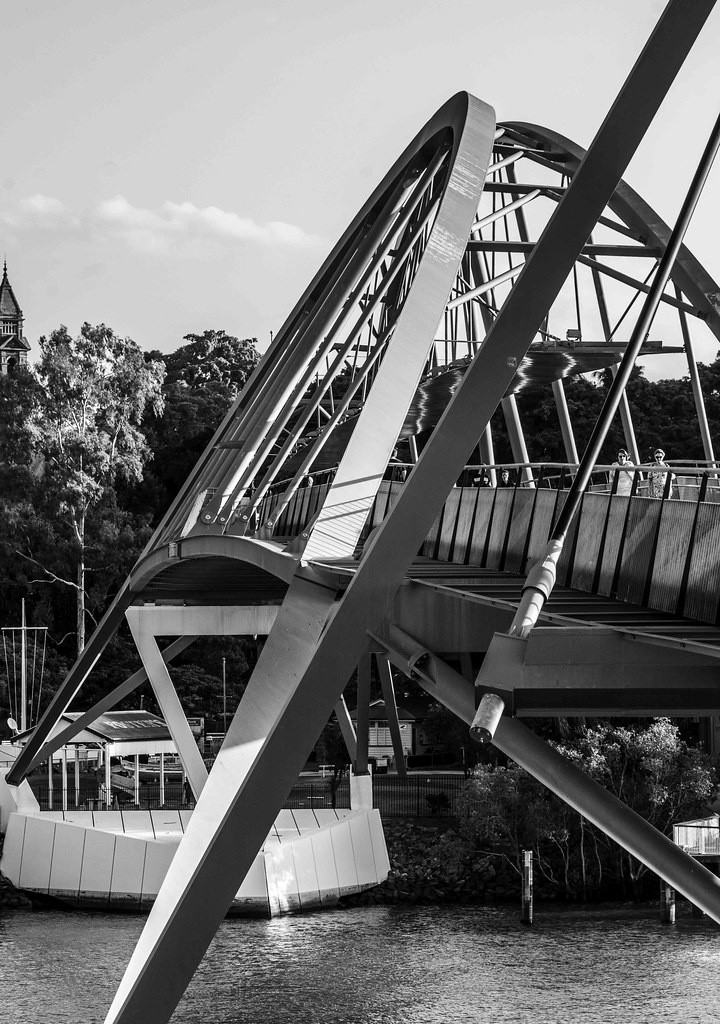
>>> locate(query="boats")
[25,759,184,802]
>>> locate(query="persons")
[609,449,638,494]
[184,776,192,805]
[647,449,675,499]
[305,472,313,487]
[384,449,407,482]
[501,470,514,487]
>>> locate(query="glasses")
[655,456,662,459]
[619,455,626,458]
